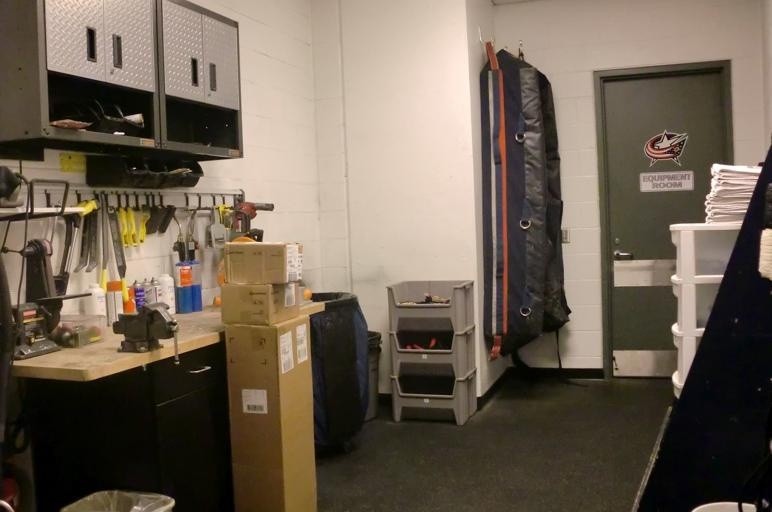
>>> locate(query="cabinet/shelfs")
[156,0,243,159]
[0,1,162,149]
[385,281,478,427]
[669,220,742,399]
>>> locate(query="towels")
[705,163,763,223]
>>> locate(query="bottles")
[106,260,203,326]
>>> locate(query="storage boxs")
[221,242,318,512]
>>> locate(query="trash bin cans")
[310,292,382,456]
[59,490,176,512]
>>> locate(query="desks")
[10,302,326,512]
